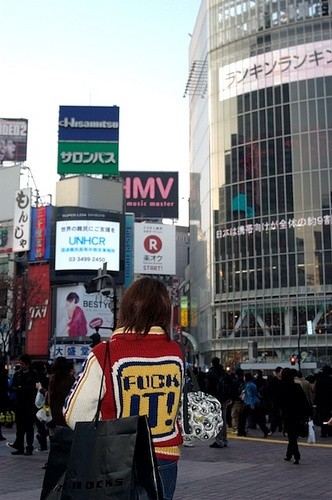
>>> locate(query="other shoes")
[262,428,270,438]
[183,440,194,446]
[293,460,299,464]
[231,430,238,435]
[26,446,33,456]
[284,456,291,461]
[209,442,223,448]
[237,431,246,437]
[36,448,48,452]
[6,442,17,450]
[223,444,228,448]
[11,447,25,455]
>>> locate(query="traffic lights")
[291,357,295,365]
[90,332,101,351]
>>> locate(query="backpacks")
[209,367,231,398]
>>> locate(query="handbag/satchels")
[299,423,309,438]
[308,418,316,444]
[175,360,222,442]
[35,390,52,423]
[39,340,165,500]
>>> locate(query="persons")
[187,365,206,391]
[59,291,87,336]
[295,364,332,438]
[205,357,233,448]
[62,276,186,500]
[0,353,54,456]
[229,367,288,438]
[5,140,16,159]
[275,369,309,465]
[41,356,76,470]
[0,140,6,161]
